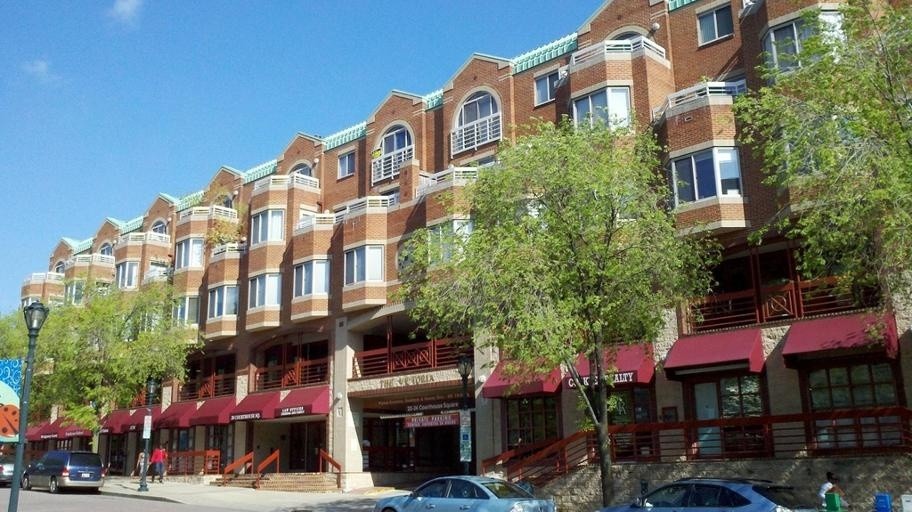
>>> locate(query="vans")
[22,450,105,494]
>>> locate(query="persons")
[816,471,854,512]
[135,448,150,480]
[150,442,168,485]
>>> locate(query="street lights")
[137,377,157,491]
[456,356,474,474]
[8,299,49,512]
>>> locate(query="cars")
[595,476,818,512]
[0,455,31,487]
[373,475,556,512]
